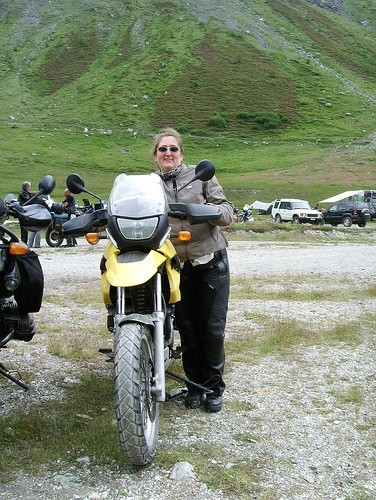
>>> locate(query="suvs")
[270,199,323,225]
[321,201,370,227]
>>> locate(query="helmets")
[22,182,31,189]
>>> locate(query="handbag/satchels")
[3,244,44,312]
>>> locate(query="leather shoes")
[186,391,202,409]
[206,393,222,412]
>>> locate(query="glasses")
[157,147,181,152]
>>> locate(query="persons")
[22,192,51,248]
[314,204,319,209]
[18,181,33,245]
[152,127,233,411]
[62,188,78,247]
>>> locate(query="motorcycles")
[46,160,223,466]
[0,174,56,391]
[237,204,254,225]
